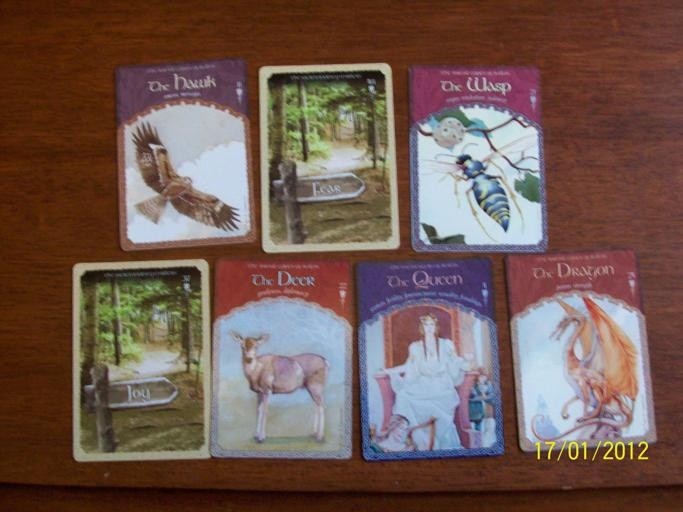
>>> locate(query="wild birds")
[530,297,639,442]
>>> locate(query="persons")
[383,311,471,452]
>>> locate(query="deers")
[228,329,330,443]
